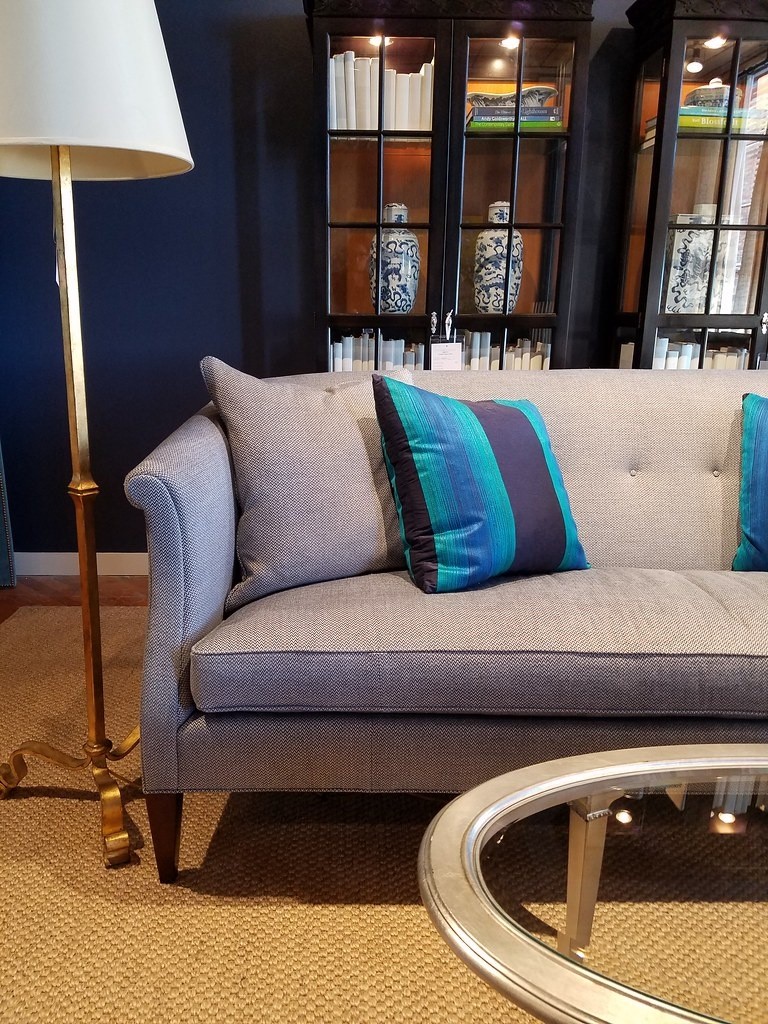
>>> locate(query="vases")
[369,203,421,314]
[472,200,520,316]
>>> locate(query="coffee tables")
[418,742,768,1024]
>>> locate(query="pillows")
[731,393,768,572]
[372,373,590,594]
[201,357,407,618]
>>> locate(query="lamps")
[687,39,703,73]
[0,0,195,867]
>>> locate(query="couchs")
[121,368,768,883]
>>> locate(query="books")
[327,49,435,142]
[652,335,750,372]
[466,105,563,131]
[330,327,551,372]
[640,107,768,152]
